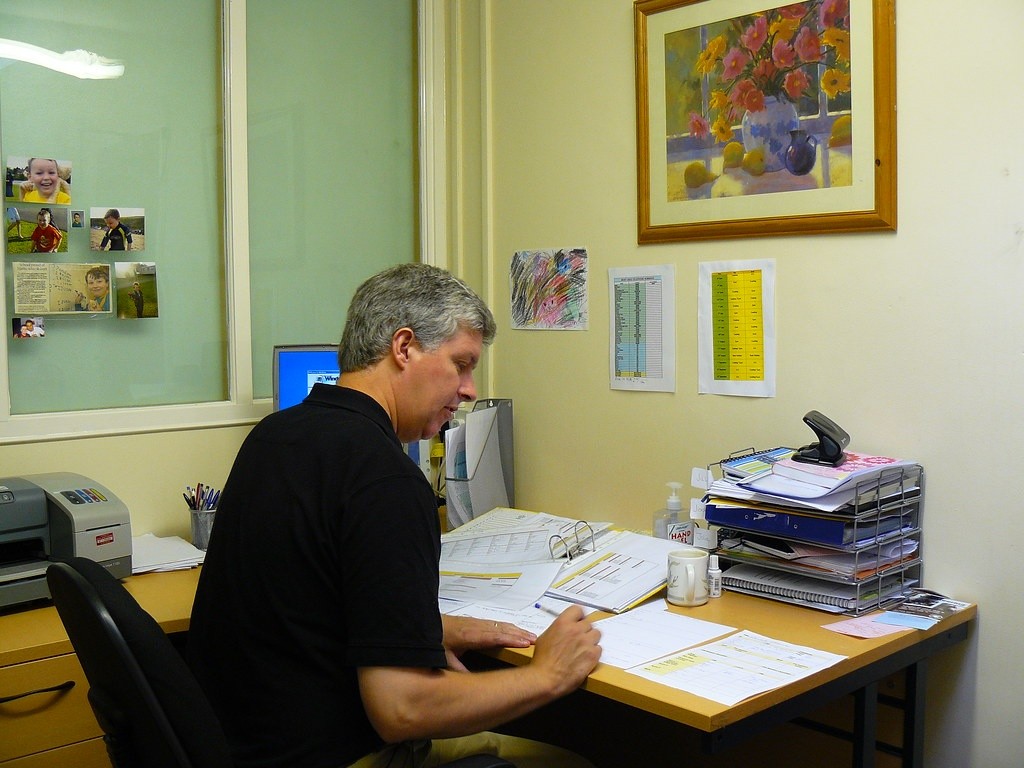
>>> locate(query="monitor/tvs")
[272,346,432,486]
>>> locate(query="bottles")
[710,553,722,599]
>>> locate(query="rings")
[494,621,497,627]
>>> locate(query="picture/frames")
[633,0,899,247]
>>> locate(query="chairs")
[47,557,518,768]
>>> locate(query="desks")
[439,502,976,768]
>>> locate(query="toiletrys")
[652,481,694,547]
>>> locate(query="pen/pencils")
[183,483,221,549]
[534,602,562,618]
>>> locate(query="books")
[771,444,899,490]
[721,564,910,609]
[740,535,798,561]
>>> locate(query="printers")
[0,472,132,611]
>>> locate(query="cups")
[188,508,215,551]
[666,549,710,608]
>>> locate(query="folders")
[706,502,912,547]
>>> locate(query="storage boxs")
[445,398,513,532]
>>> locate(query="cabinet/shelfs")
[0,559,205,768]
[703,446,923,618]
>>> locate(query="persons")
[23,170,29,181]
[14,320,45,337]
[99,209,133,251]
[128,282,144,318]
[31,208,63,253]
[19,157,71,204]
[72,213,82,227]
[185,263,602,768]
[6,171,15,197]
[75,266,110,311]
[6,208,24,239]
